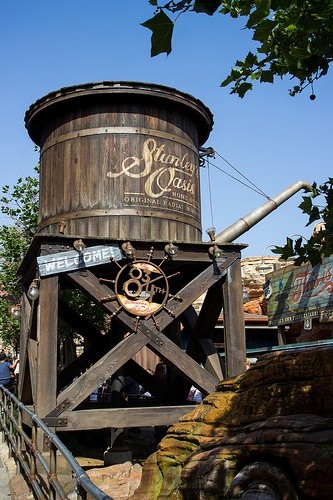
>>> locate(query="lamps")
[209,245,222,257]
[164,243,178,254]
[73,239,85,252]
[122,242,134,255]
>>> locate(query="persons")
[0,352,20,409]
[110,363,166,407]
